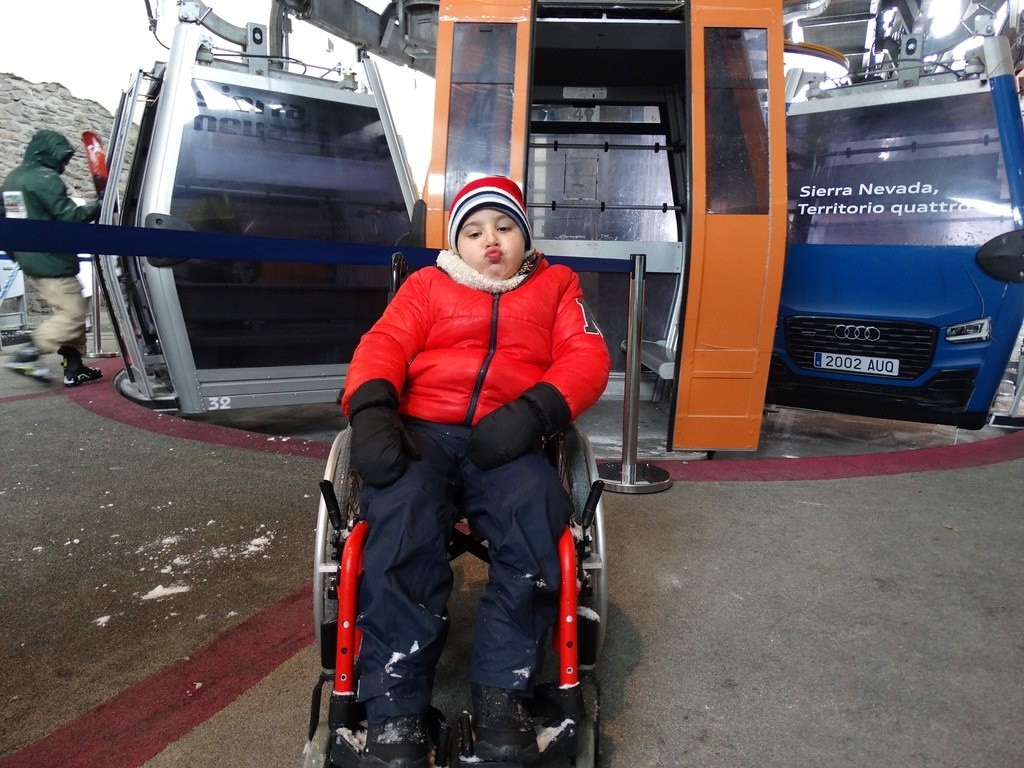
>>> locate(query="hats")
[448,176,532,259]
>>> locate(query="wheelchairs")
[301,252,606,768]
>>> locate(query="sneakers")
[470,684,540,764]
[363,713,430,768]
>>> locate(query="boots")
[57,346,103,388]
[10,343,50,377]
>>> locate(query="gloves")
[463,395,546,471]
[348,403,422,490]
[96,187,105,201]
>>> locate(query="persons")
[343,178,610,768]
[0,130,106,386]
